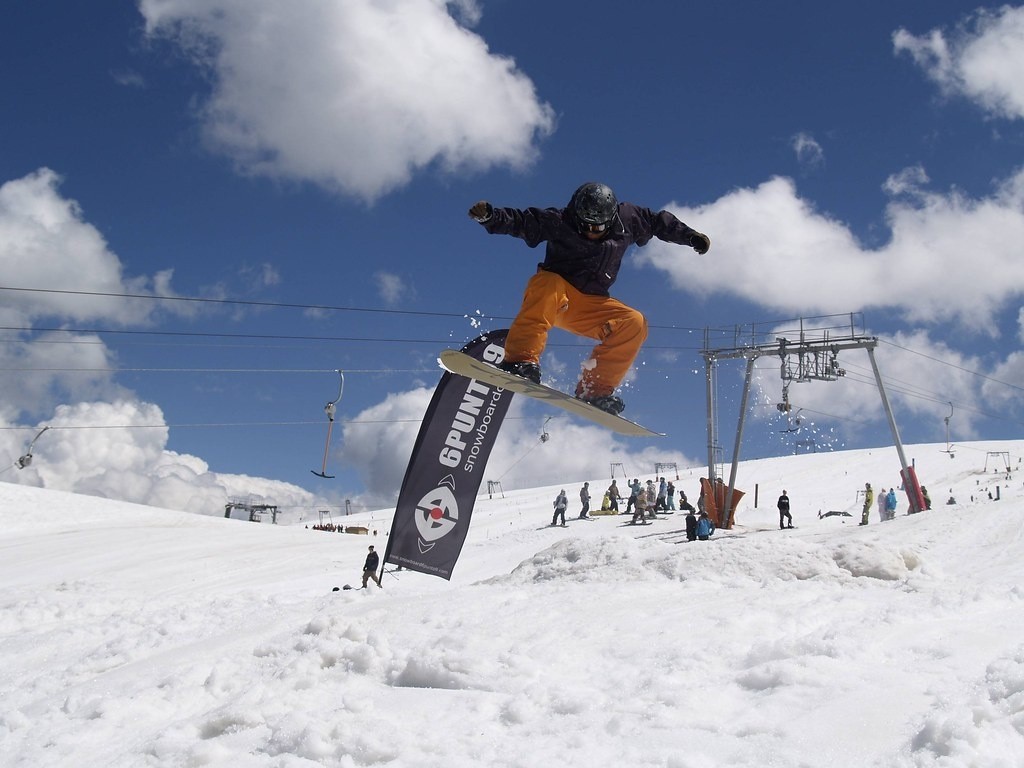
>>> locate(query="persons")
[624,478,657,524]
[655,477,725,515]
[860,483,873,525]
[306,524,347,533]
[386,532,388,537]
[777,490,794,529]
[878,488,897,522]
[373,530,377,536]
[362,545,382,588]
[685,510,715,541]
[921,486,931,510]
[397,564,411,572]
[601,480,621,511]
[551,489,568,525]
[950,458,1024,501]
[580,482,591,518]
[468,182,710,415]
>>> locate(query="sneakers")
[588,395,624,415]
[503,360,541,383]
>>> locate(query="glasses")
[577,221,610,234]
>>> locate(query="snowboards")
[438,347,668,438]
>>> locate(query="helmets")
[572,183,618,238]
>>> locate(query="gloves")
[468,202,493,223]
[691,232,710,254]
